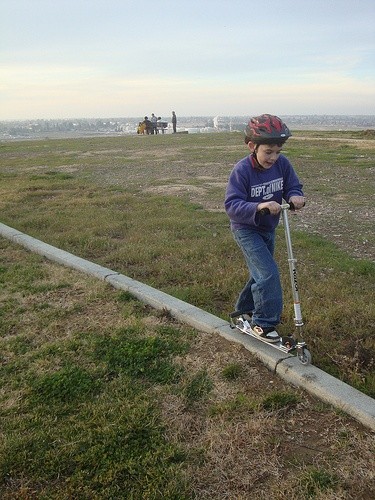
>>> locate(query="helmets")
[244,114,291,143]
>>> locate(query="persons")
[136,110,178,136]
[221,114,307,344]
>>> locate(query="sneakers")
[237,312,252,327]
[250,322,280,343]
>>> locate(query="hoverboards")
[231,201,312,367]
